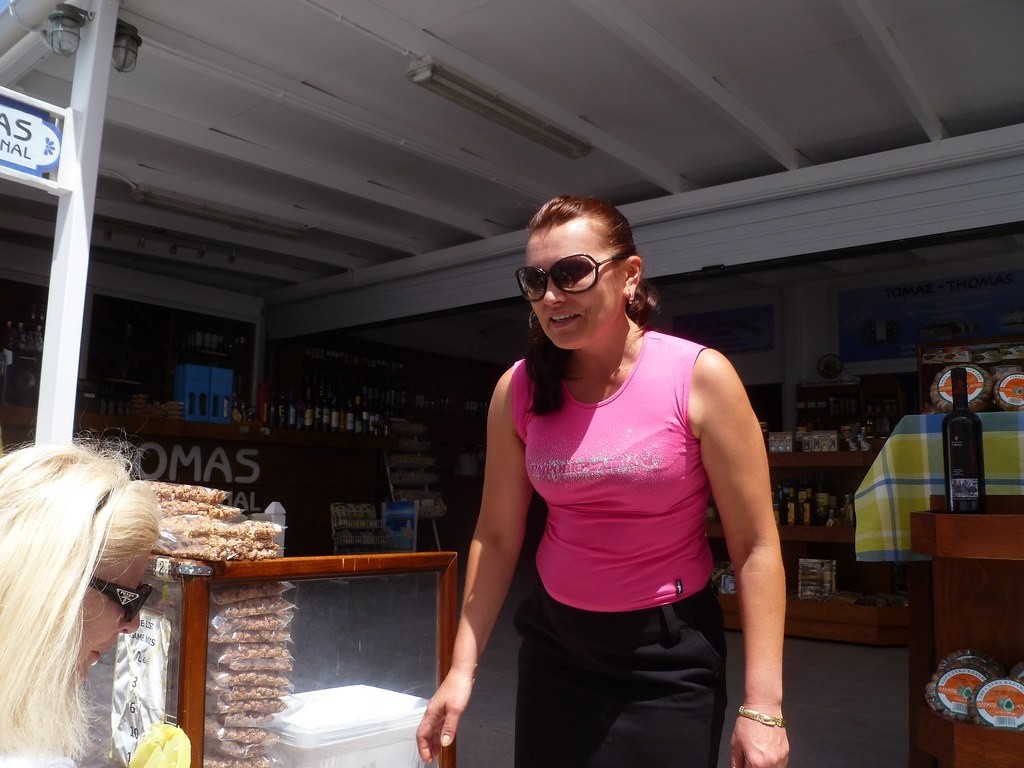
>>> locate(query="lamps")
[404,55,591,160]
[197,240,206,258]
[229,247,236,264]
[111,18,142,73]
[136,232,144,248]
[103,230,112,241]
[48,3,94,57]
[130,189,311,239]
[169,239,177,253]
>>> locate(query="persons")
[0,429,160,768]
[417,192,790,768]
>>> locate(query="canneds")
[181,330,224,349]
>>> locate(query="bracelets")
[738,705,786,728]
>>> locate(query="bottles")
[100,383,131,416]
[178,330,487,437]
[770,478,854,527]
[941,367,986,513]
[5,302,45,354]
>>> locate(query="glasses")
[88,576,153,622]
[514,253,636,302]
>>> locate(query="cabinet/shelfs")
[906,496,1023,767]
[86,293,256,403]
[330,501,387,554]
[707,450,906,646]
[383,436,449,552]
[274,334,507,428]
[795,384,908,450]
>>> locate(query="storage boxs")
[266,684,429,768]
